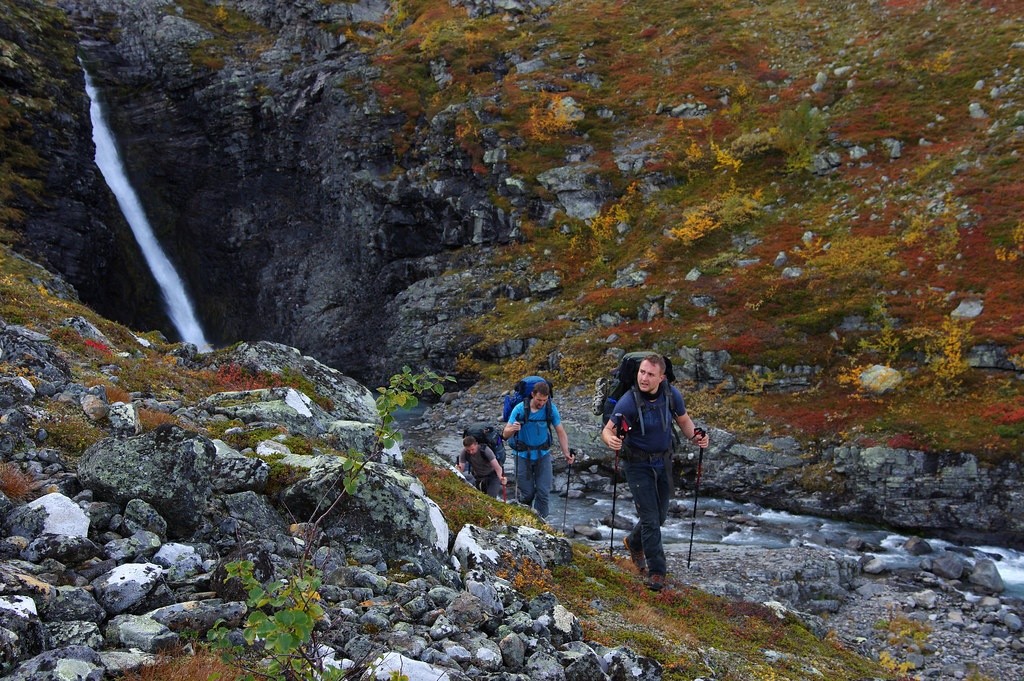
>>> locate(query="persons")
[601,355,709,593]
[500,380,574,520]
[454,435,509,500]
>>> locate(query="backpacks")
[503,375,554,429]
[463,426,506,468]
[602,351,676,441]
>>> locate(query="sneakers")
[646,574,665,591]
[623,536,646,571]
